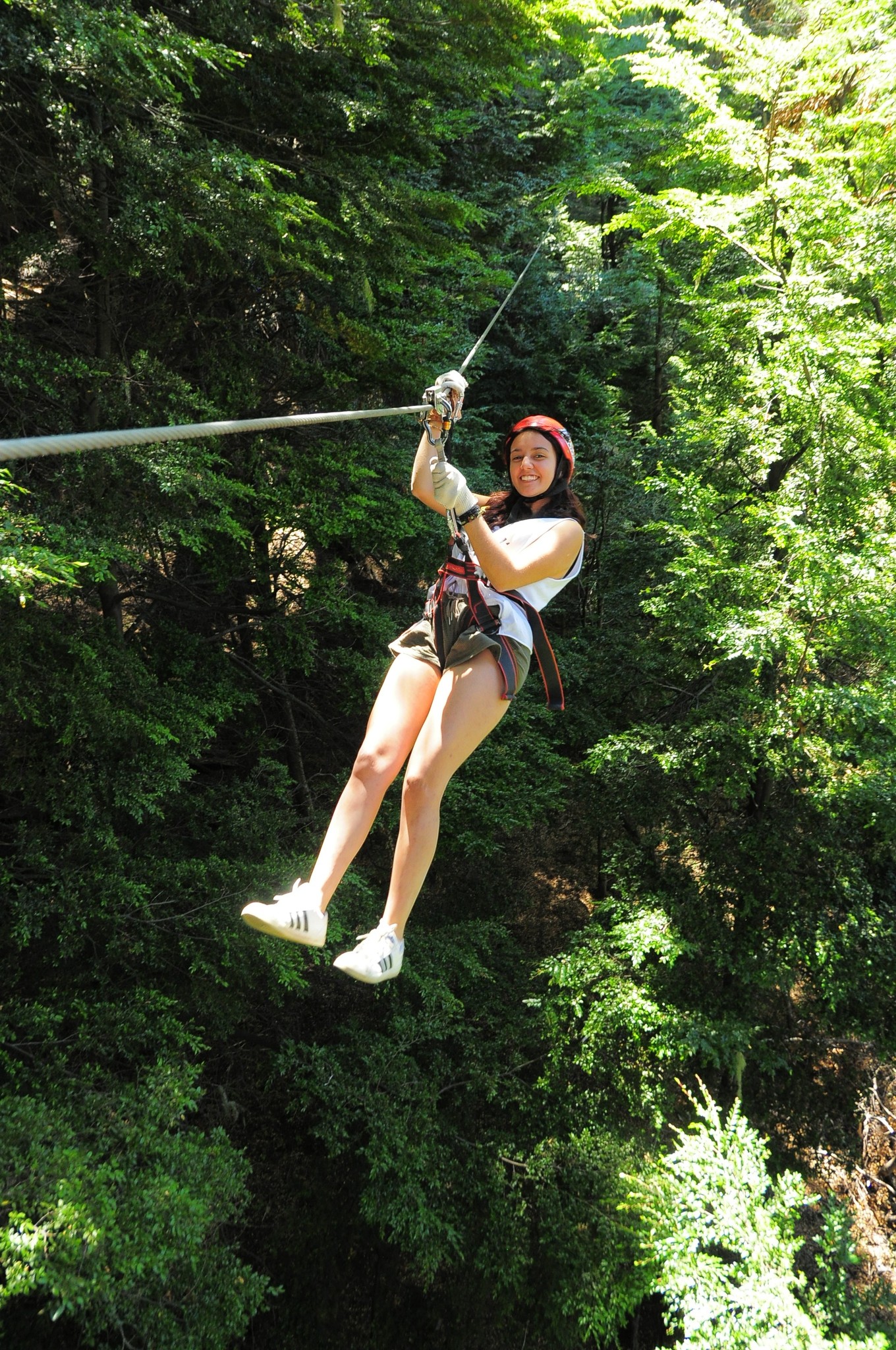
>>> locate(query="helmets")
[504,415,576,485]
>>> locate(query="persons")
[241,371,584,985]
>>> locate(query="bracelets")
[458,505,481,525]
[428,425,443,432]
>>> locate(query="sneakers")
[332,918,405,984]
[241,878,329,948]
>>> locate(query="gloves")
[429,456,479,516]
[435,370,468,423]
